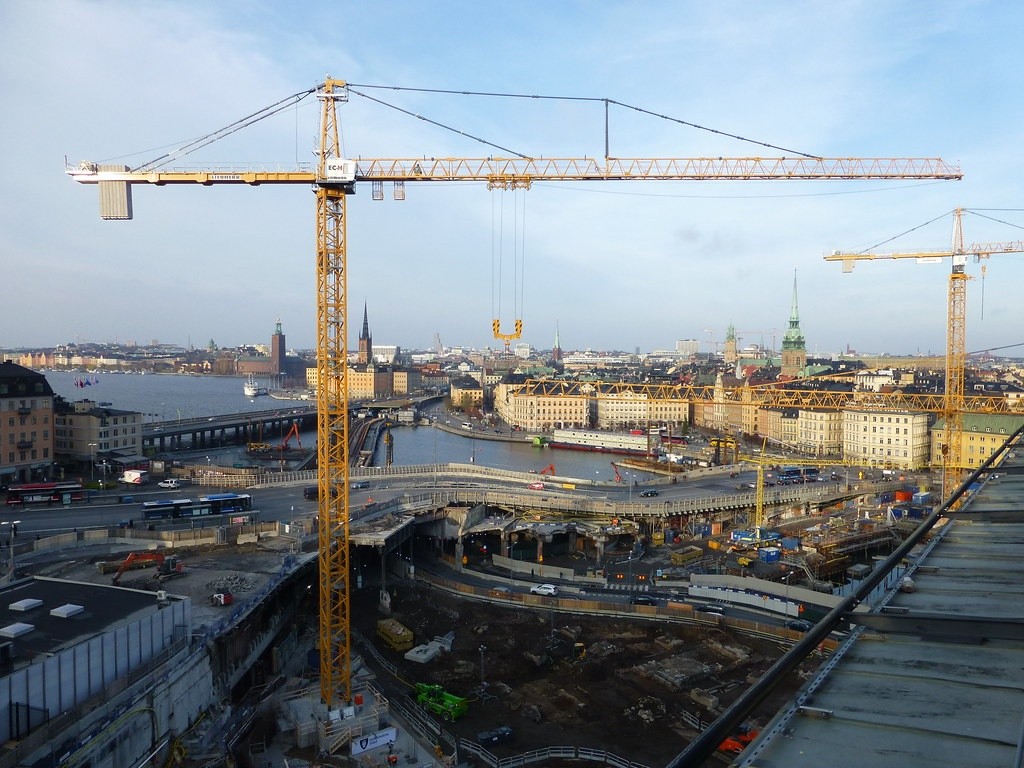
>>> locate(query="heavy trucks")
[246,441,272,453]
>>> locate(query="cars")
[638,488,658,498]
[736,473,843,490]
[304,486,339,500]
[784,618,813,631]
[626,594,657,606]
[157,478,181,490]
[529,583,558,596]
[697,604,725,614]
[351,480,370,489]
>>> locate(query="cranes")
[514,359,1024,513]
[822,207,1022,518]
[69,72,967,709]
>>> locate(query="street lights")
[782,570,795,632]
[625,471,637,502]
[506,540,518,594]
[627,550,633,603]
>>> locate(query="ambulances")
[117,469,150,484]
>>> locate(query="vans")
[493,586,512,594]
[526,481,544,489]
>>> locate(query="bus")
[7,480,84,505]
[777,467,820,479]
[142,491,253,521]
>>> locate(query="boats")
[244,373,267,396]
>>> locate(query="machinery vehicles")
[112,551,189,584]
[276,422,305,453]
